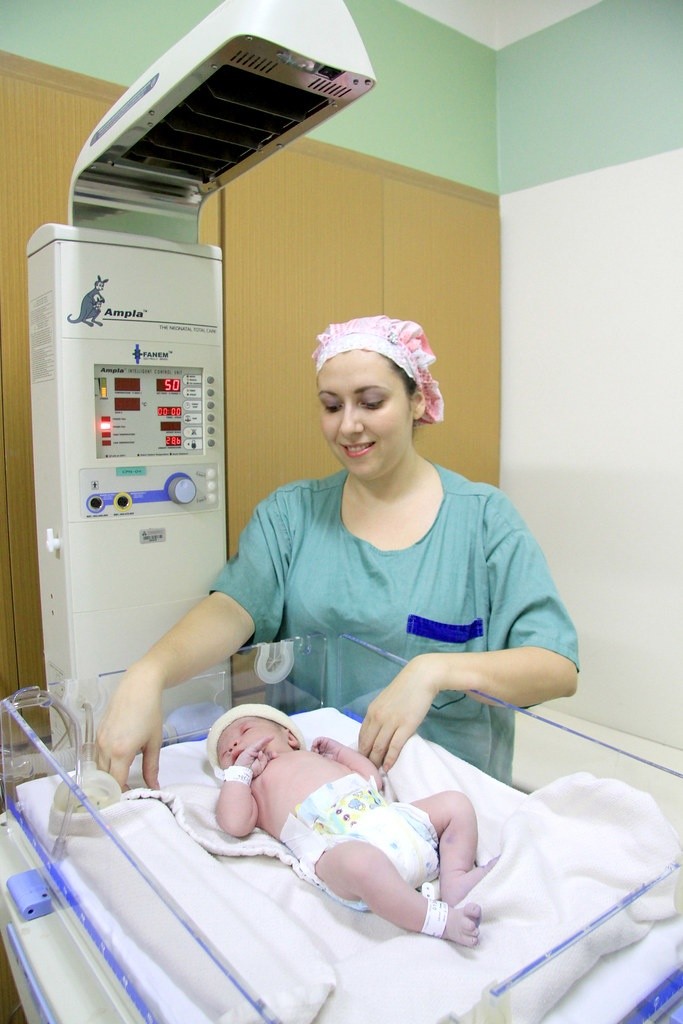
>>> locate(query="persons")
[94,316,579,794]
[207,703,503,948]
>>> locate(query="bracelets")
[214,766,253,785]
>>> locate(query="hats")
[205,703,305,776]
[313,314,444,425]
